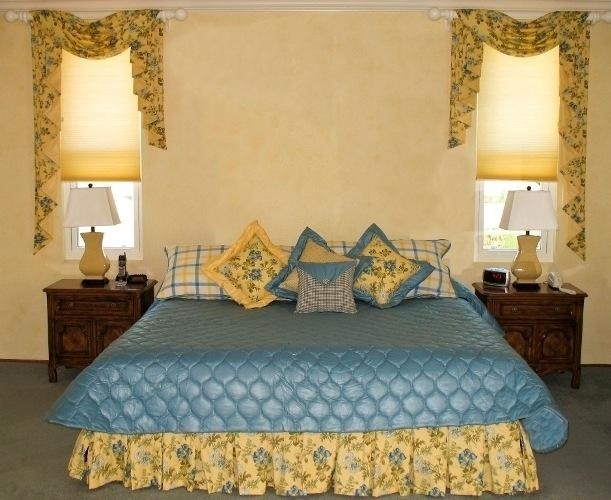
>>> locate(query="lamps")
[62,182,120,282]
[497,189,557,289]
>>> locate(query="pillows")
[326,237,458,300]
[152,243,298,304]
[340,222,437,311]
[199,220,292,309]
[265,225,372,305]
[292,258,359,316]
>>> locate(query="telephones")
[547,271,564,288]
[115,252,129,287]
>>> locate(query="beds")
[40,233,570,500]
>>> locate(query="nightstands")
[473,281,588,389]
[41,275,160,381]
